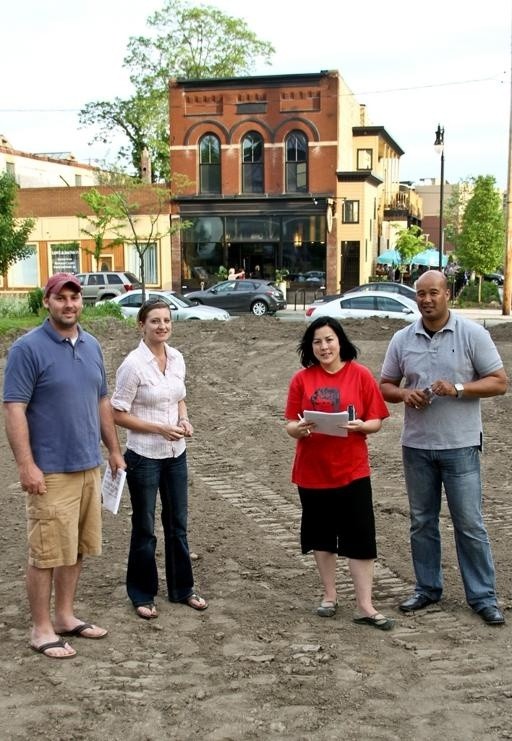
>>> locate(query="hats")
[43,273,82,297]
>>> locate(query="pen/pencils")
[297,413,310,434]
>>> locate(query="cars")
[466,269,505,286]
[287,270,326,282]
[306,291,423,322]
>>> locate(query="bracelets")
[178,415,189,422]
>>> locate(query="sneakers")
[353,606,395,630]
[317,597,339,617]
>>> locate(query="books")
[303,410,348,438]
[100,465,127,516]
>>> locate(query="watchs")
[453,383,464,401]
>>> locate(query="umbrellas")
[377,249,402,264]
[411,249,449,268]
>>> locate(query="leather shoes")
[476,606,505,623]
[399,594,440,612]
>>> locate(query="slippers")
[28,634,78,659]
[180,593,208,610]
[136,604,160,618]
[55,623,108,639]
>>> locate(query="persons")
[227,266,261,281]
[376,264,425,282]
[109,298,208,618]
[3,273,128,661]
[380,270,507,624]
[285,317,392,630]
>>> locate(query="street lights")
[433,123,447,270]
